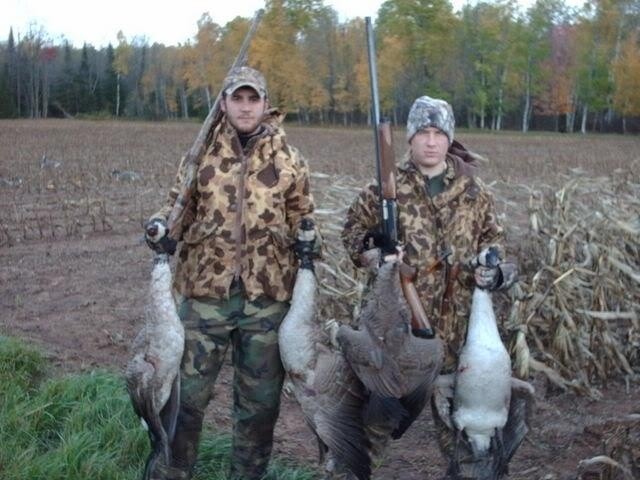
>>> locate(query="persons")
[341,92,508,480]
[145,66,323,480]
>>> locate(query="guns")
[163,8,266,245]
[365,17,434,338]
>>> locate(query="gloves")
[362,232,407,263]
[145,218,177,255]
[474,257,502,290]
[289,229,323,260]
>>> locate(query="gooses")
[124,217,184,479]
[276,214,445,480]
[433,242,535,480]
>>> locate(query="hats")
[406,95,455,148]
[222,67,268,98]
